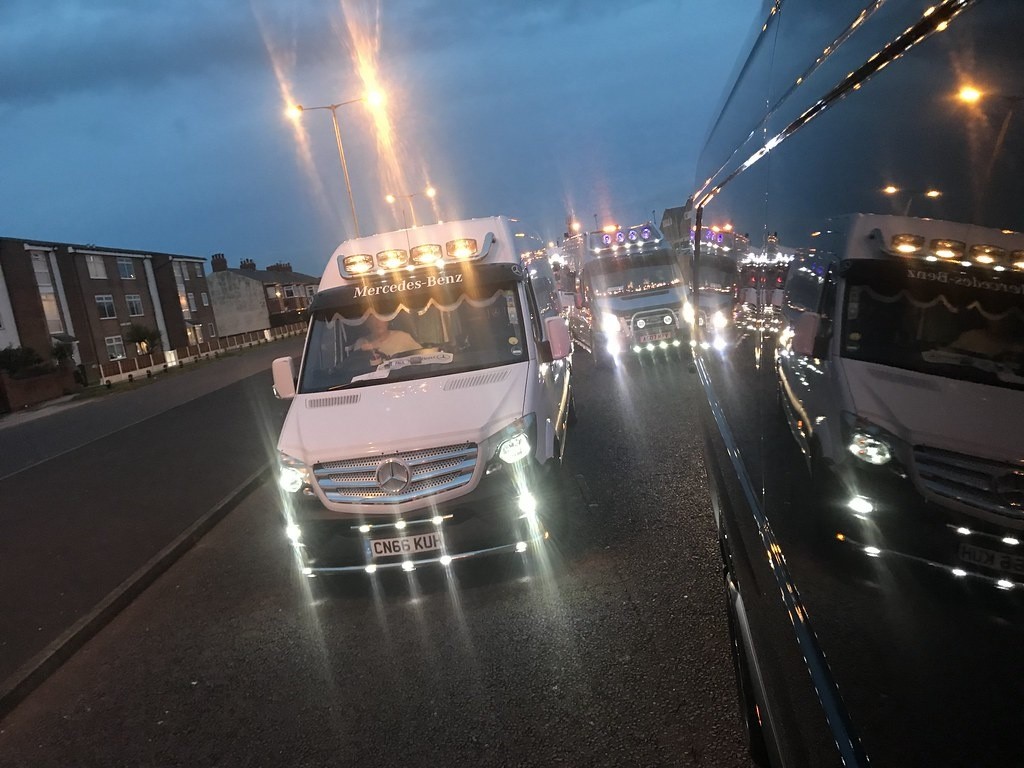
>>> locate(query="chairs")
[346,315,417,346]
[460,296,516,353]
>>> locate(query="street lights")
[883,185,943,217]
[291,90,387,238]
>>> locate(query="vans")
[553,221,695,364]
[777,218,1023,618]
[272,216,570,576]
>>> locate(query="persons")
[350,313,423,355]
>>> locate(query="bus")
[662,1,1024,767]
